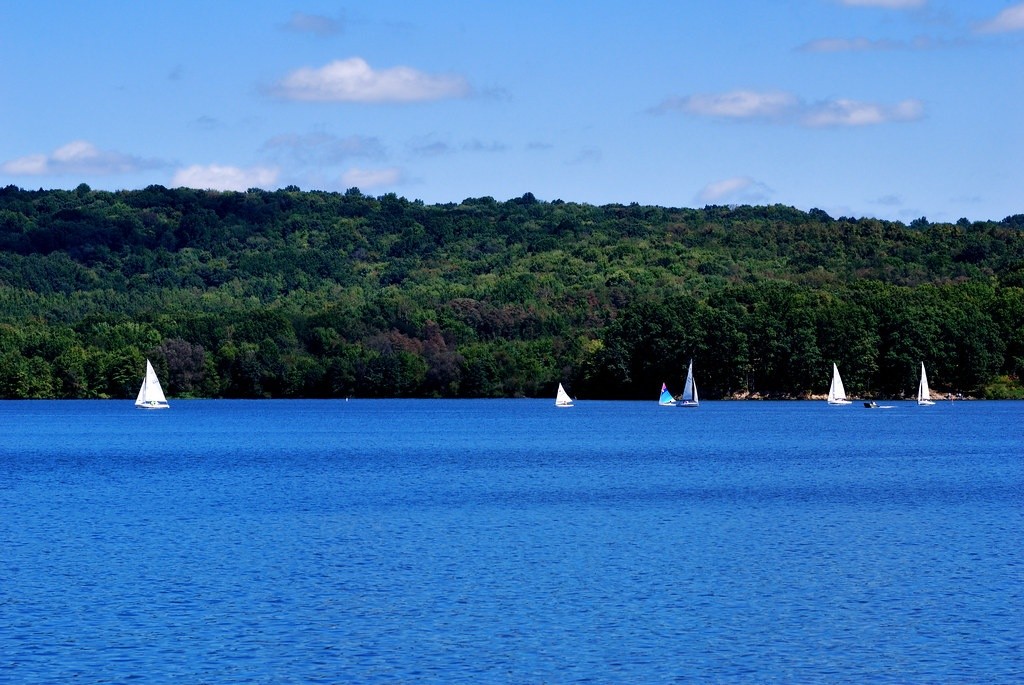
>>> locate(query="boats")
[864,403,880,408]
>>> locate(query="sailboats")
[827,362,853,405]
[135,359,169,409]
[916,360,936,405]
[659,382,677,407]
[675,358,700,408]
[555,382,575,408]
